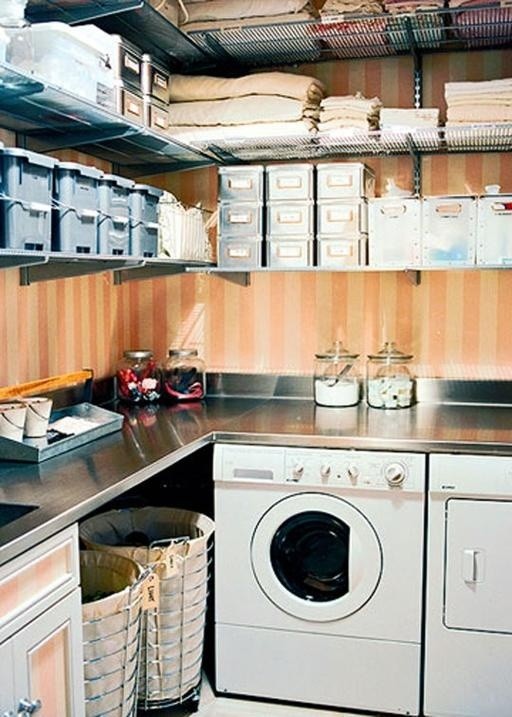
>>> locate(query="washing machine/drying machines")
[213,442,426,717]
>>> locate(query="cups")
[0,396,54,443]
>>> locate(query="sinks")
[0,501,40,530]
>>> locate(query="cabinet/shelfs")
[215,17,511,287]
[1,521,84,717]
[0,1,219,282]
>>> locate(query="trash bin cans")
[80,550,158,717]
[78,506,216,717]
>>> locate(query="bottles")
[160,347,206,402]
[365,342,418,409]
[117,399,207,449]
[313,401,415,442]
[313,340,363,407]
[114,348,163,406]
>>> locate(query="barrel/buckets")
[79,506,216,710]
[0,402,27,443]
[80,550,152,717]
[21,397,53,437]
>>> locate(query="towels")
[441,76,512,144]
[317,91,442,148]
[163,0,318,156]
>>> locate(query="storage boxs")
[217,158,512,269]
[2,145,160,262]
[104,30,171,135]
